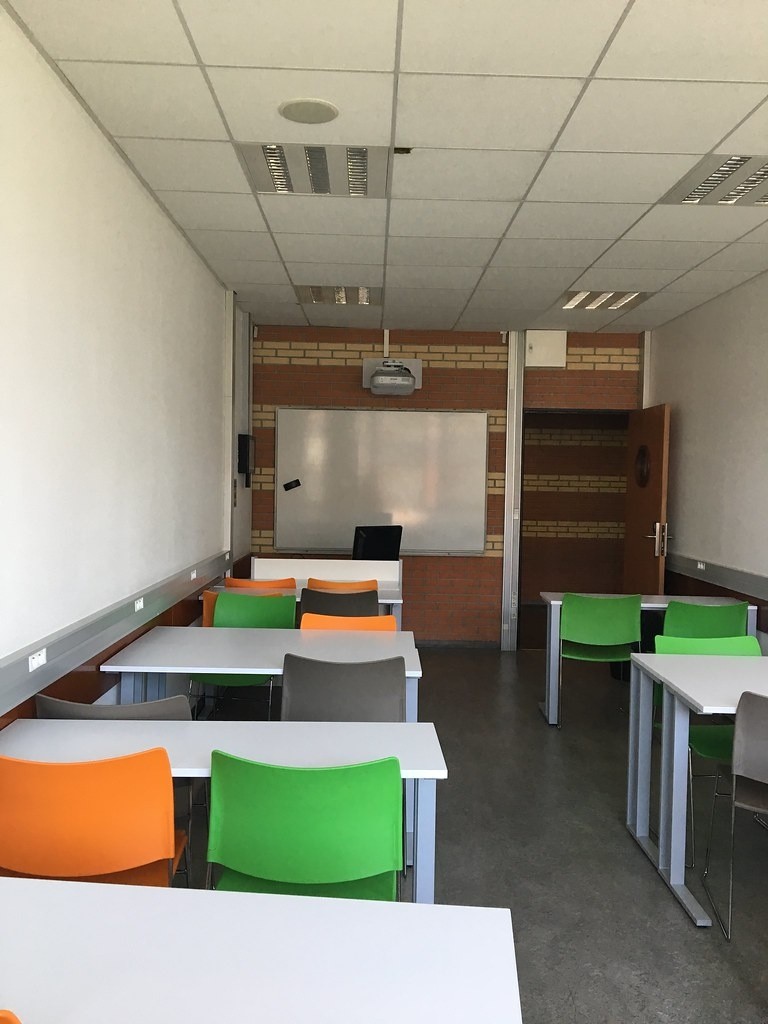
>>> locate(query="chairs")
[187,591,297,722]
[654,633,763,870]
[35,692,209,848]
[558,593,642,729]
[203,590,283,627]
[225,577,297,589]
[700,690,768,943]
[651,600,749,723]
[306,578,379,590]
[0,747,190,888]
[300,612,397,631]
[299,588,379,617]
[281,652,409,879]
[205,749,404,902]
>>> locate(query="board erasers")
[282,479,301,491]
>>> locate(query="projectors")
[371,370,415,396]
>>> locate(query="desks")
[98,626,424,867]
[0,718,448,904]
[198,586,403,631]
[0,878,525,1024]
[540,591,758,726]
[216,579,400,615]
[626,652,768,927]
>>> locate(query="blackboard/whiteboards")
[272,407,489,558]
